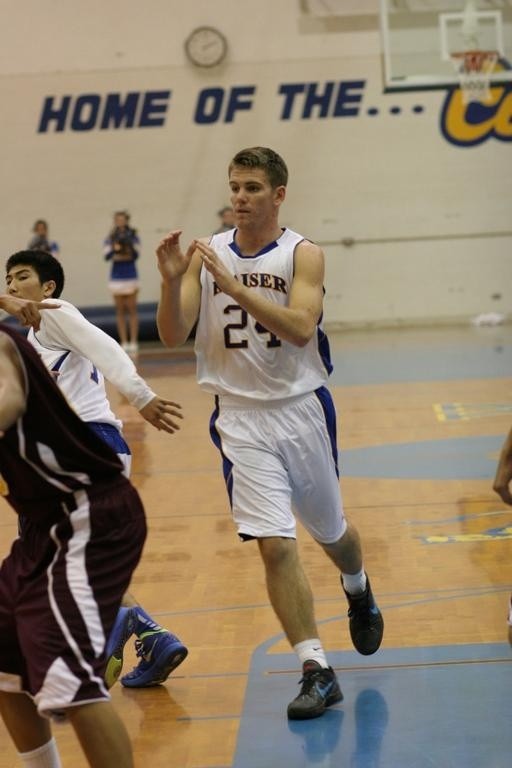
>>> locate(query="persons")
[1,290,64,335]
[24,220,62,264]
[2,247,190,693]
[155,144,384,720]
[101,209,143,353]
[212,206,237,238]
[2,319,148,767]
[492,425,512,507]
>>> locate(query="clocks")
[184,27,228,69]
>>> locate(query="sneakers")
[287,660,343,720]
[339,569,384,656]
[102,606,139,690]
[121,627,188,688]
